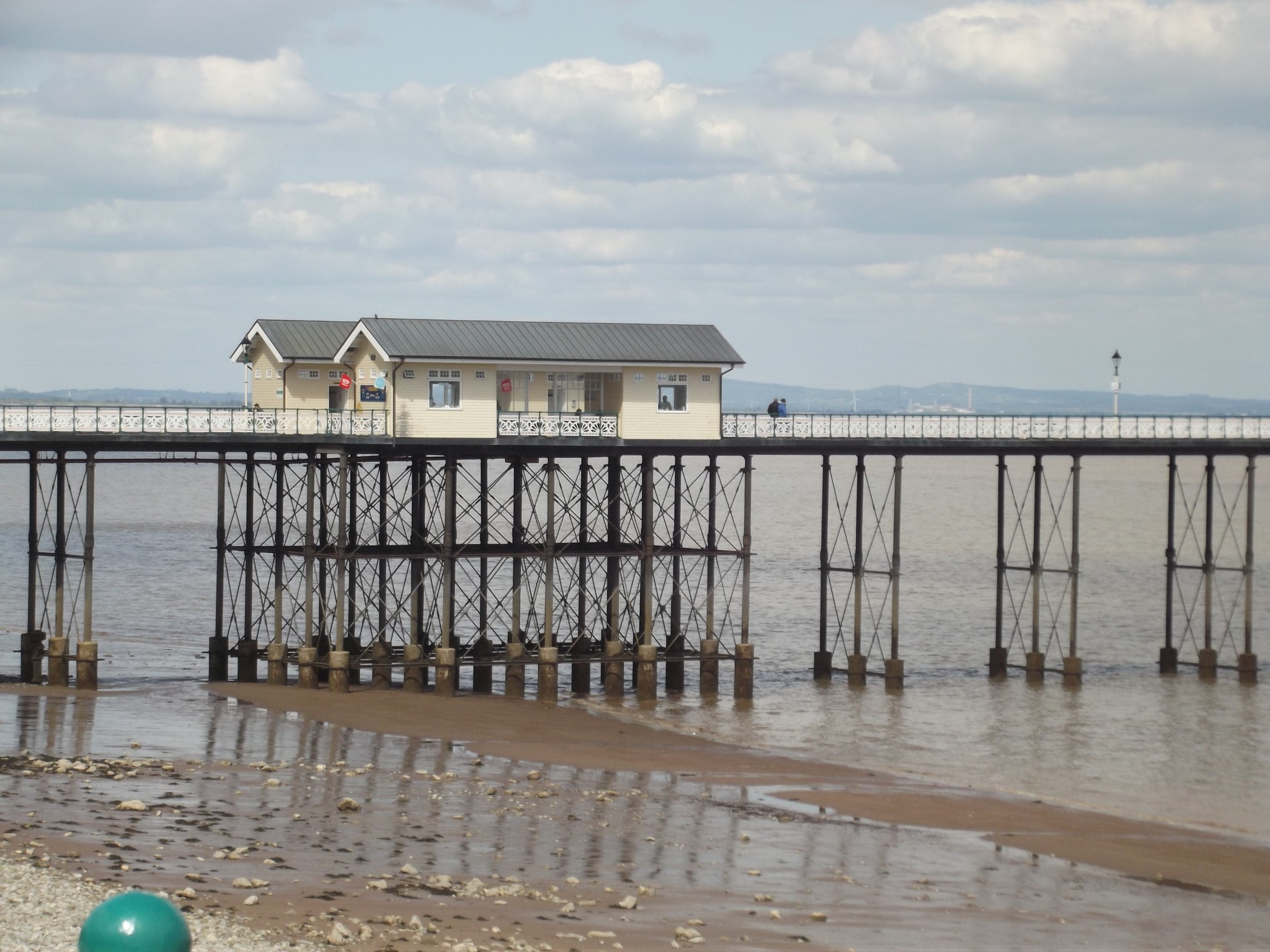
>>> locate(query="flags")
[339,374,351,389]
[501,379,512,393]
[374,371,386,390]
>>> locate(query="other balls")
[75,890,196,952]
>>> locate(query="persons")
[779,399,788,417]
[768,398,780,417]
[659,395,672,410]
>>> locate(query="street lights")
[1111,347,1122,415]
[241,333,253,412]
